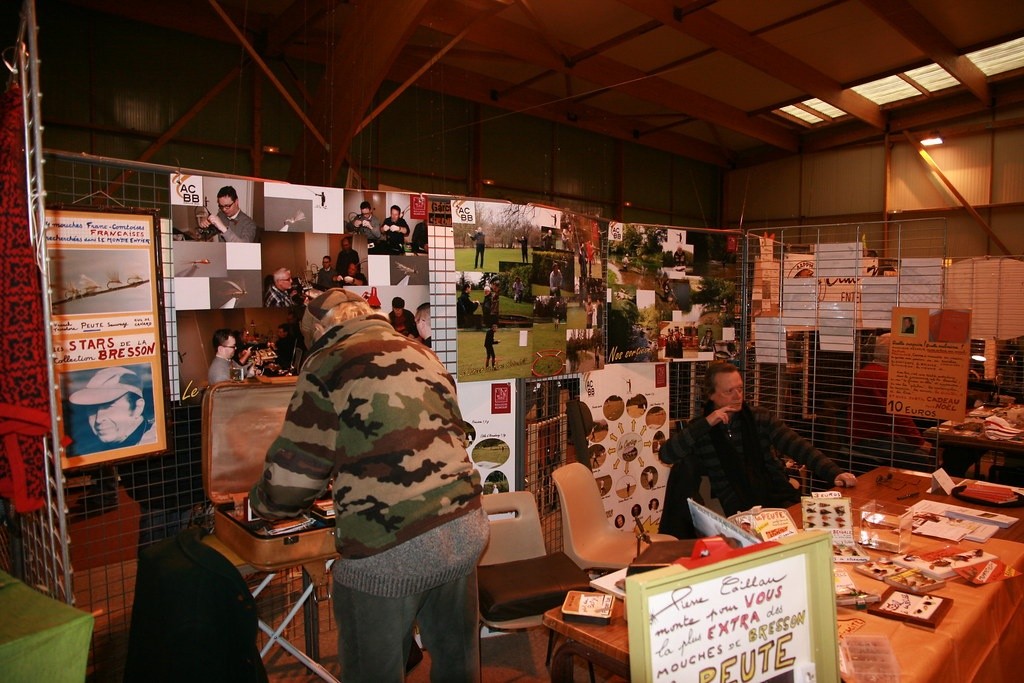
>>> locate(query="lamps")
[920,130,944,147]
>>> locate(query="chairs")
[469,490,555,682]
[552,462,680,577]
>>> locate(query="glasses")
[221,345,237,350]
[218,202,234,209]
[361,213,370,215]
[278,278,290,281]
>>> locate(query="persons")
[200,186,256,242]
[590,425,661,528]
[617,230,716,357]
[248,287,489,683]
[902,317,914,333]
[346,201,428,254]
[456,225,601,371]
[659,362,857,517]
[847,333,933,471]
[264,238,366,306]
[208,322,295,386]
[389,297,431,347]
[68,367,156,447]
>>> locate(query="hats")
[301,289,366,333]
[68,367,143,405]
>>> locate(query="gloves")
[383,224,390,232]
[389,225,399,232]
[362,220,373,229]
[354,220,362,227]
[344,276,353,283]
[336,275,343,281]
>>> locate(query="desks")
[203,534,341,682]
[540,467,1024,683]
[923,406,1024,485]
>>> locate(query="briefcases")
[200,374,337,570]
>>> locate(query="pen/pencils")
[897,492,919,500]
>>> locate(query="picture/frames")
[863,585,955,629]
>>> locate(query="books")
[313,499,334,516]
